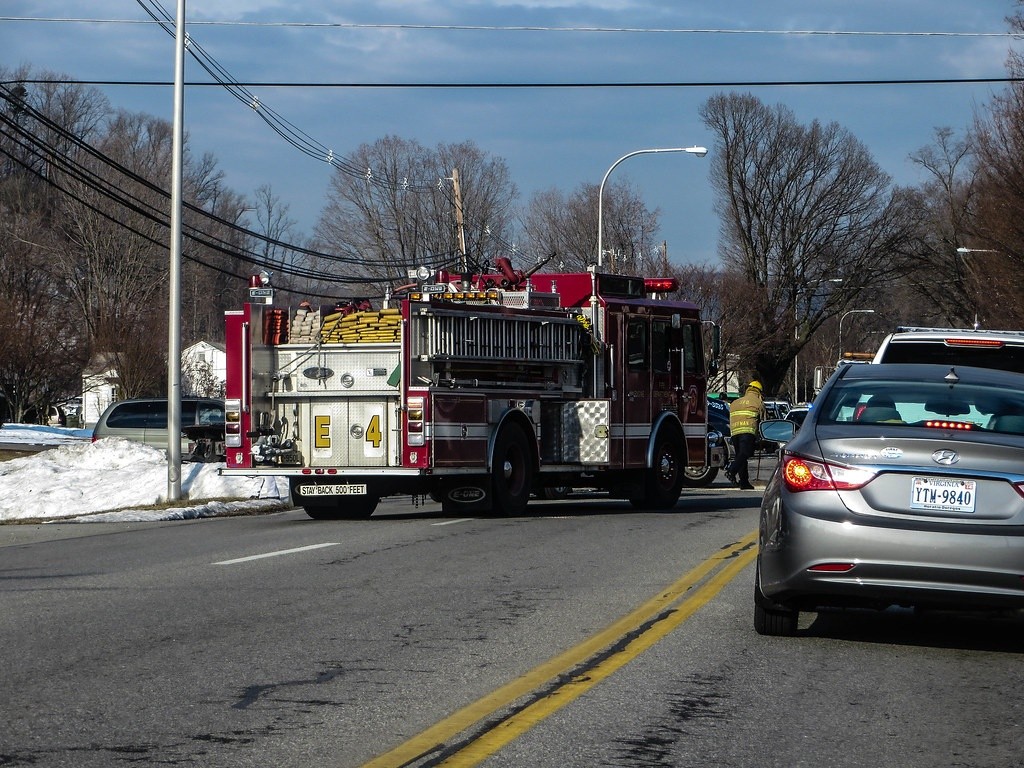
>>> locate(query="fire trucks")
[224,256,722,520]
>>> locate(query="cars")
[751,360,1024,638]
[780,408,810,448]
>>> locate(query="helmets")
[748,380,763,391]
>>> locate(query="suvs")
[871,332,1024,374]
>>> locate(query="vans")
[91,396,226,459]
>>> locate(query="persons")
[866,393,896,408]
[680,385,707,424]
[778,403,788,418]
[718,393,730,409]
[724,380,767,489]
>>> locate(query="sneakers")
[739,479,754,490]
[724,469,738,487]
